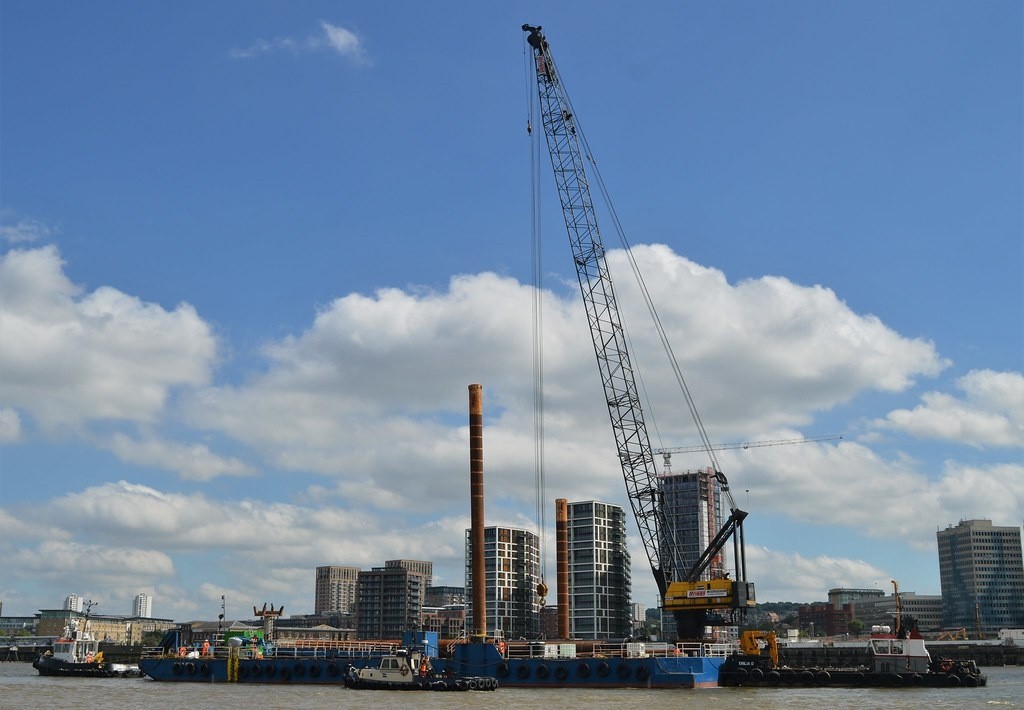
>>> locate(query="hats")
[205,639,208,642]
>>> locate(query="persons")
[498,639,506,656]
[202,639,210,656]
[253,634,266,656]
[420,660,428,676]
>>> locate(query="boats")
[134,381,729,687]
[32,599,144,679]
[716,578,989,688]
[343,621,499,691]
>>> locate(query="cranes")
[615,432,844,473]
[517,19,761,641]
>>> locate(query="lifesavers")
[940,658,954,672]
[498,641,506,655]
[909,673,925,688]
[399,664,410,677]
[460,678,499,691]
[238,660,339,681]
[889,674,905,688]
[495,662,649,682]
[945,674,978,688]
[172,661,210,677]
[734,667,832,687]
